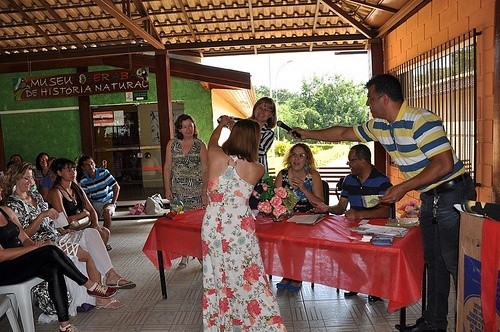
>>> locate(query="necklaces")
[58,182,77,206]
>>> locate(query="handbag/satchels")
[144,193,164,215]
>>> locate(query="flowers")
[252,174,287,218]
[398,201,421,219]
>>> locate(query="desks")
[142,207,426,332]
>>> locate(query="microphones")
[277,120,301,138]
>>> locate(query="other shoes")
[106,244,112,251]
[395,317,446,332]
[368,295,382,303]
[344,291,357,298]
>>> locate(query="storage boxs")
[456,211,500,332]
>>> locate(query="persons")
[217,97,277,209]
[312,145,391,302]
[275,143,324,292]
[48,159,110,245]
[0,152,56,202]
[0,176,117,332]
[79,156,120,251]
[288,75,477,332]
[0,162,136,309]
[164,114,208,269]
[201,116,288,332]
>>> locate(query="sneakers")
[60,324,80,332]
[86,283,118,298]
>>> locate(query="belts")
[425,172,468,196]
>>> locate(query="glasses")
[290,151,306,160]
[256,104,271,113]
[348,158,359,162]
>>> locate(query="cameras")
[371,238,390,245]
[372,234,394,242]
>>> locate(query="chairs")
[0,277,44,332]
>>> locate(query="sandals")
[177,256,188,270]
[288,281,303,292]
[276,278,289,289]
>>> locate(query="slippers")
[95,298,125,310]
[105,277,136,289]
